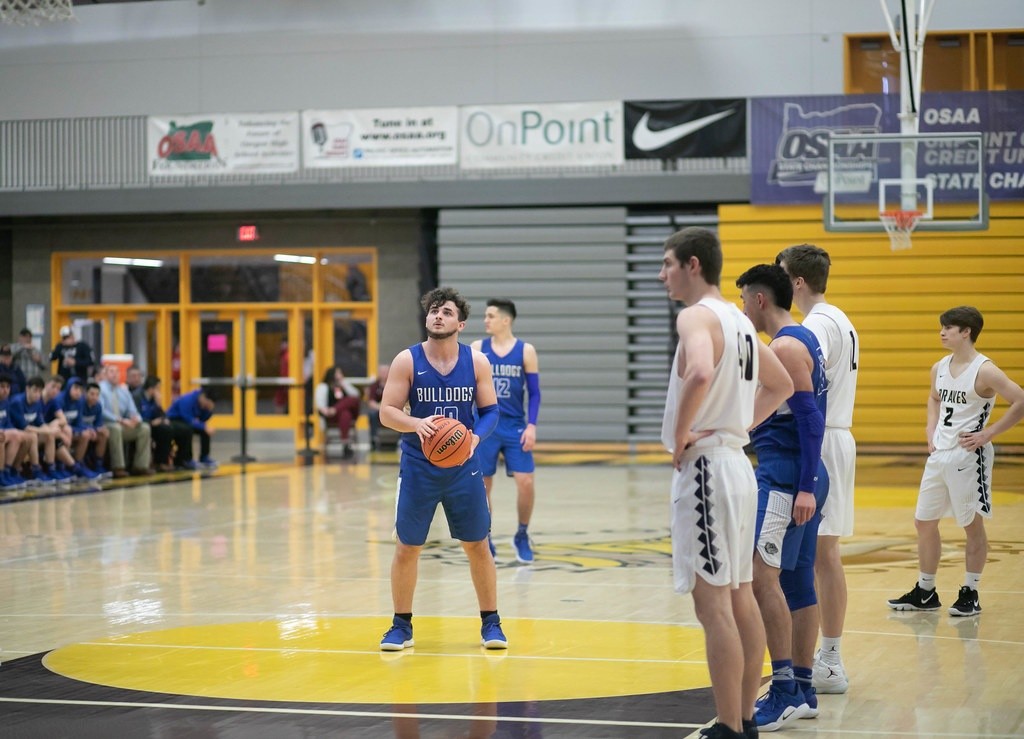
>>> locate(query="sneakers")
[754,686,819,719]
[379,617,415,651]
[0,455,114,490]
[480,614,507,649]
[488,533,497,561]
[753,682,810,732]
[947,584,983,617]
[698,714,760,739]
[886,581,942,611]
[512,531,535,564]
[811,648,849,694]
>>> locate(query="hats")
[59,324,70,337]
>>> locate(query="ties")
[111,387,120,416]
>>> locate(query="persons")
[316,365,361,459]
[887,306,1024,618]
[0,326,218,491]
[367,364,403,452]
[658,227,794,739]
[774,244,860,693]
[735,265,829,733]
[469,298,541,564]
[379,288,507,651]
[273,338,313,417]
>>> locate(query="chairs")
[321,418,401,457]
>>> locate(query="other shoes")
[154,456,217,471]
[131,467,157,477]
[318,407,331,420]
[114,469,131,478]
[342,446,353,459]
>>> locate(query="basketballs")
[422,418,472,468]
